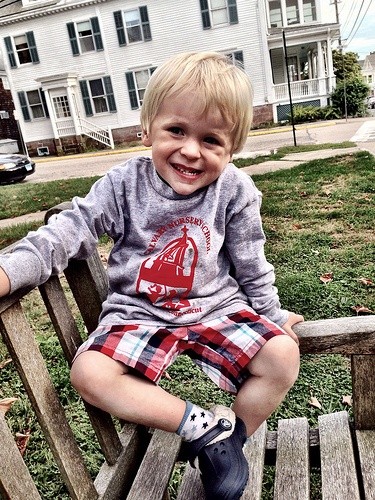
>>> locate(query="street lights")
[267,31,297,146]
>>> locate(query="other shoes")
[187,404,249,500]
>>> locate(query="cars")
[366,96,375,105]
[0,153,36,183]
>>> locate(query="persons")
[0,52,304,500]
